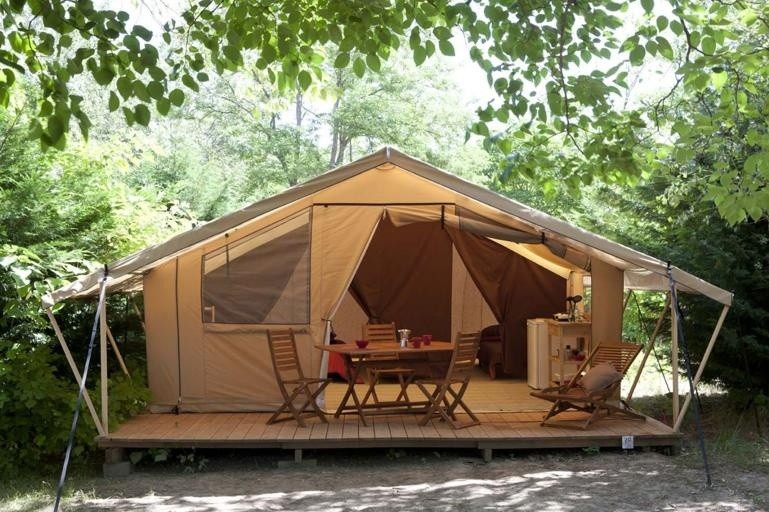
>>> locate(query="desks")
[314,341,474,426]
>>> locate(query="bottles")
[564,344,572,361]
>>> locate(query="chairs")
[361,321,416,410]
[264,326,334,428]
[529,341,646,430]
[414,329,482,430]
[477,324,504,380]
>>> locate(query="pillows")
[576,360,625,397]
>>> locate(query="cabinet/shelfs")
[547,321,592,388]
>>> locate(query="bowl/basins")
[354,339,369,349]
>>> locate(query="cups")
[411,337,421,348]
[422,335,432,345]
[400,339,408,348]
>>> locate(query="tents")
[41,148,737,512]
